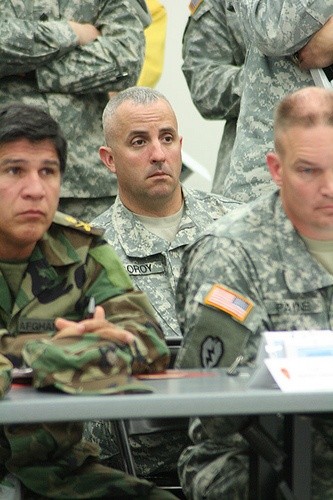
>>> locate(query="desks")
[0,365,333,500]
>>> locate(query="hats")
[22,335,155,395]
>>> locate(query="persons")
[81,87,249,485]
[0,100,176,500]
[0,0,149,225]
[175,87,333,500]
[182,1,333,201]
[135,0,167,89]
